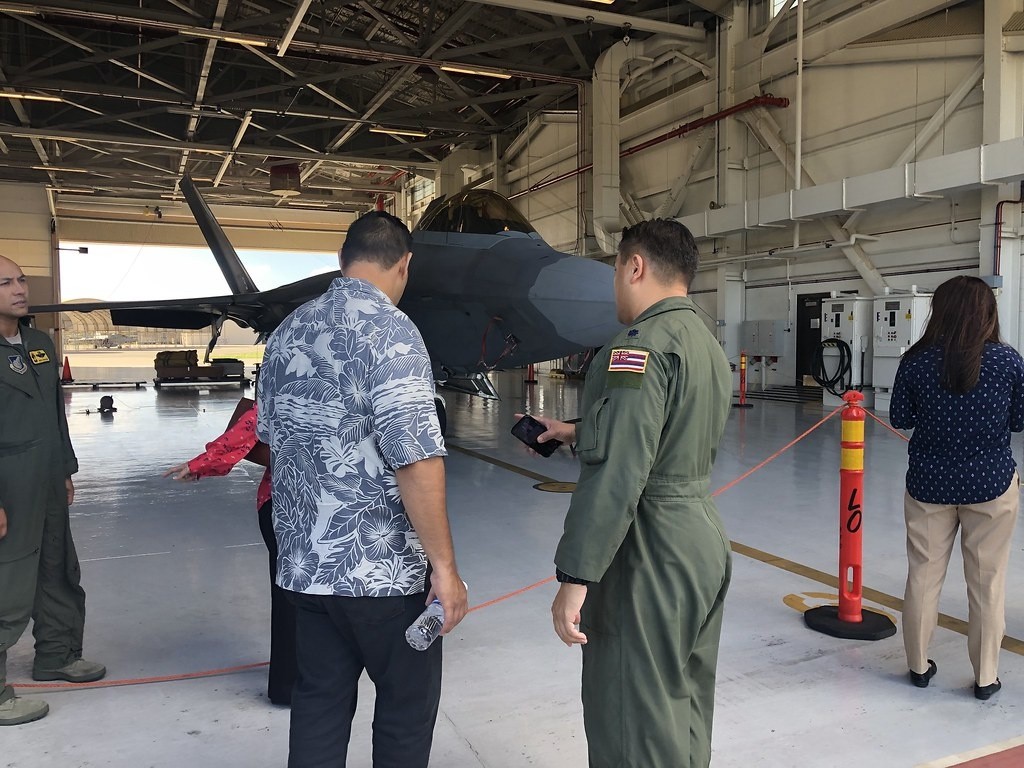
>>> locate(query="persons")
[889,276,1024,700]
[514,218,733,768]
[164,403,291,706]
[0,255,106,726]
[257,211,468,768]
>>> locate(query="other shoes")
[974,677,1001,700]
[910,659,937,688]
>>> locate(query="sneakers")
[32,657,106,683]
[0,697,49,726]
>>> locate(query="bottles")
[405,580,468,651]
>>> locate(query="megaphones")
[709,201,721,210]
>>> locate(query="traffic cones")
[525,364,538,384]
[61,356,74,382]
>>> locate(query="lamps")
[584,16,595,41]
[216,108,221,113]
[154,206,163,218]
[270,157,304,197]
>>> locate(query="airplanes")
[27,172,628,408]
[67,332,139,349]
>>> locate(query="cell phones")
[511,414,563,458]
